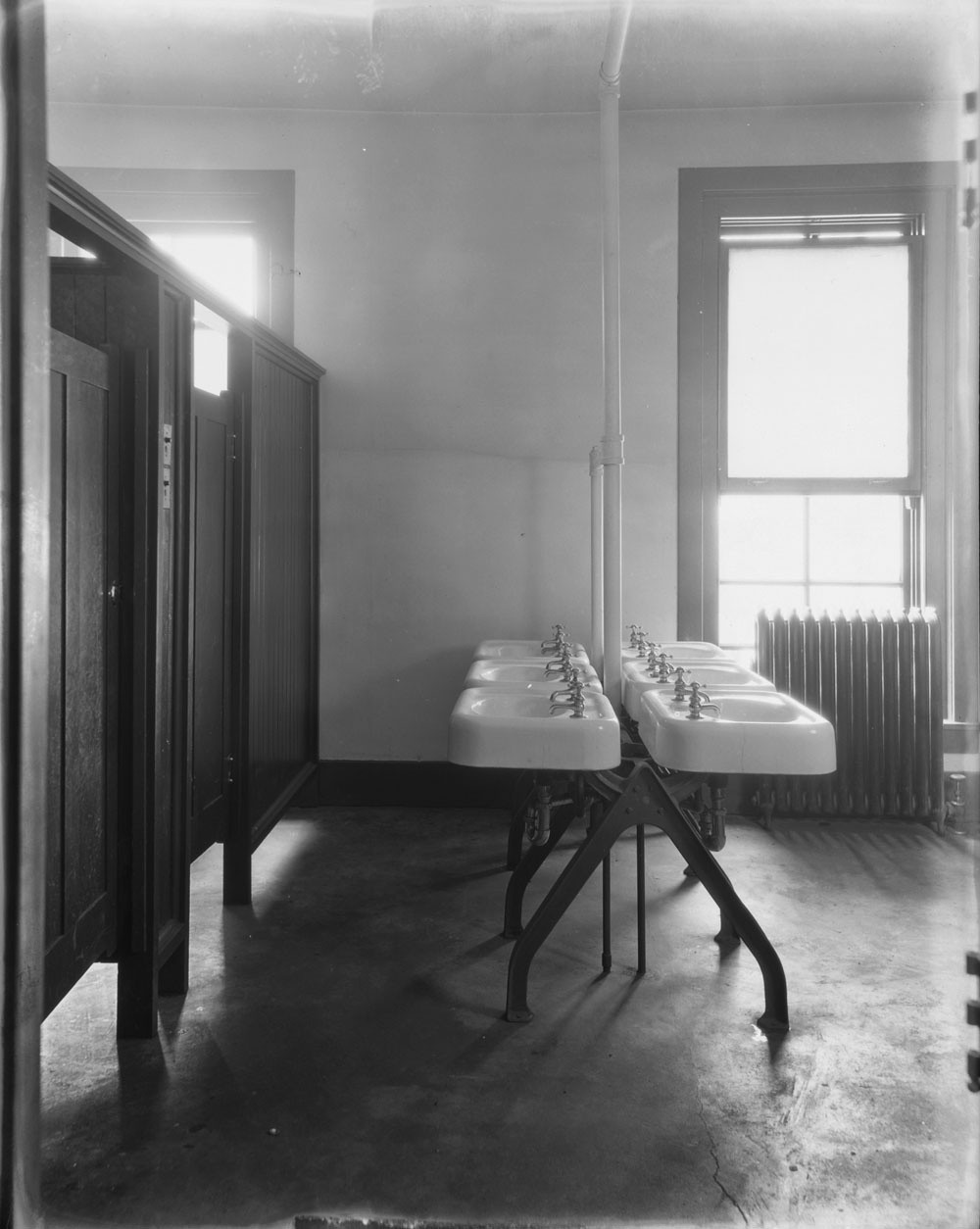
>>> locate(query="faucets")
[541,623,584,719]
[626,624,720,719]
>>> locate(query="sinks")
[470,640,590,664]
[620,641,725,662]
[448,688,621,771]
[638,689,837,776]
[463,660,603,694]
[622,660,777,721]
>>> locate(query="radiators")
[755,606,972,835]
